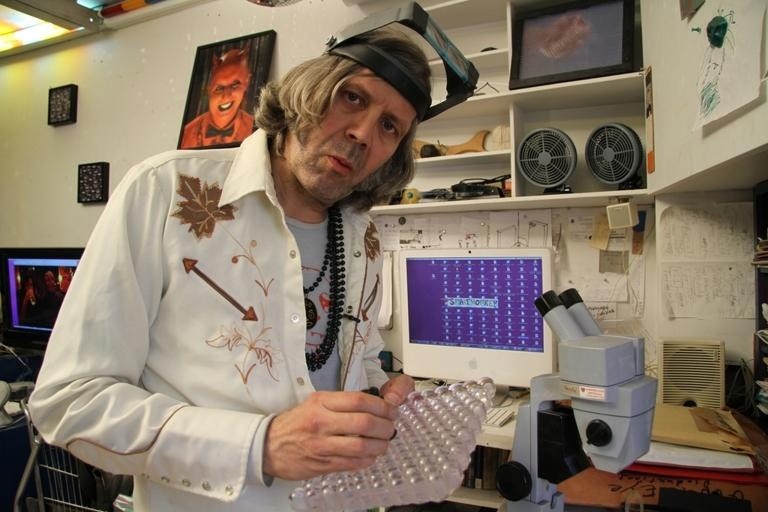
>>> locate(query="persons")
[181,49,254,148]
[18,269,64,322]
[28,31,431,512]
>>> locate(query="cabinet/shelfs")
[365,0,649,214]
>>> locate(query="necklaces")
[301,204,347,372]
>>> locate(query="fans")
[514,120,645,195]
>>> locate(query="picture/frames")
[46,83,78,126]
[175,29,277,150]
[507,0,635,94]
[76,161,109,204]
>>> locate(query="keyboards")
[481,408,514,427]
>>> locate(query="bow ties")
[205,123,233,138]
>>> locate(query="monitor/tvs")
[399,247,556,406]
[0,247,85,347]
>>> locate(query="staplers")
[448,183,503,200]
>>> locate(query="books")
[463,446,510,489]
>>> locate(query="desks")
[556,468,768,508]
[448,392,529,508]
